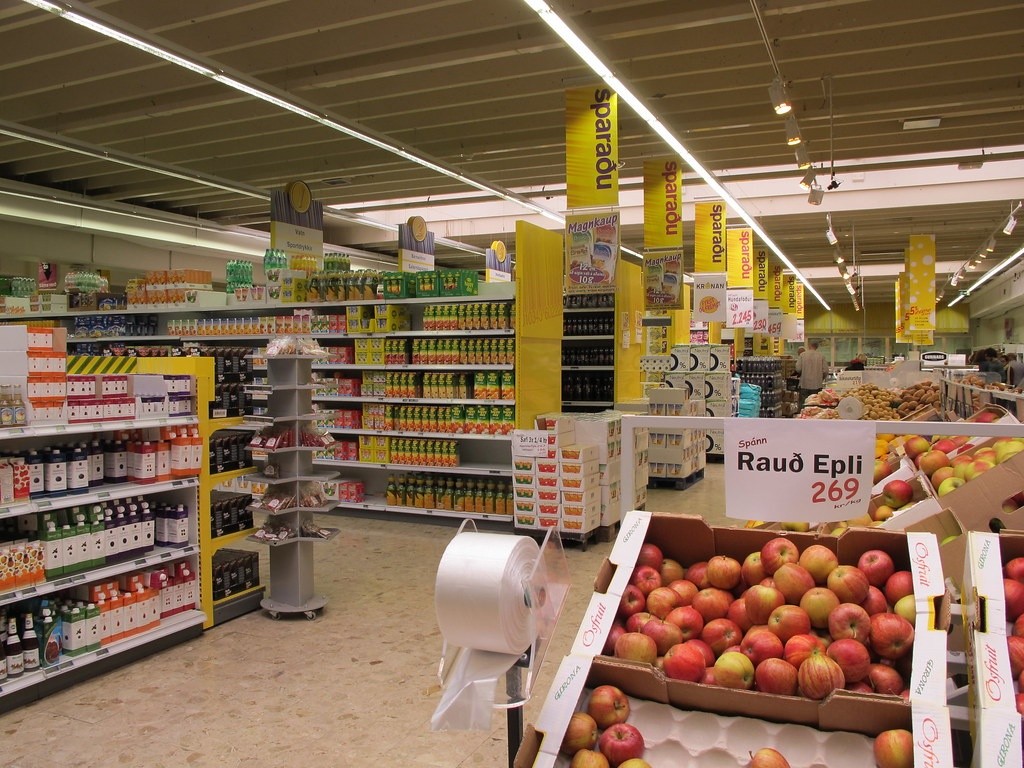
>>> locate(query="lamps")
[935,236,996,306]
[799,167,817,191]
[832,249,860,312]
[767,75,792,115]
[794,144,811,171]
[808,184,825,206]
[1003,215,1017,235]
[825,229,838,246]
[783,113,802,147]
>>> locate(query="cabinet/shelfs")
[0,219,808,720]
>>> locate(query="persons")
[1007,353,1024,387]
[1000,355,1013,386]
[791,347,805,377]
[796,341,829,414]
[845,354,866,370]
[985,348,1006,382]
[968,350,988,372]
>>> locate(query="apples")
[561,412,1024,768]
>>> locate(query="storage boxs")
[525,403,1024,768]
